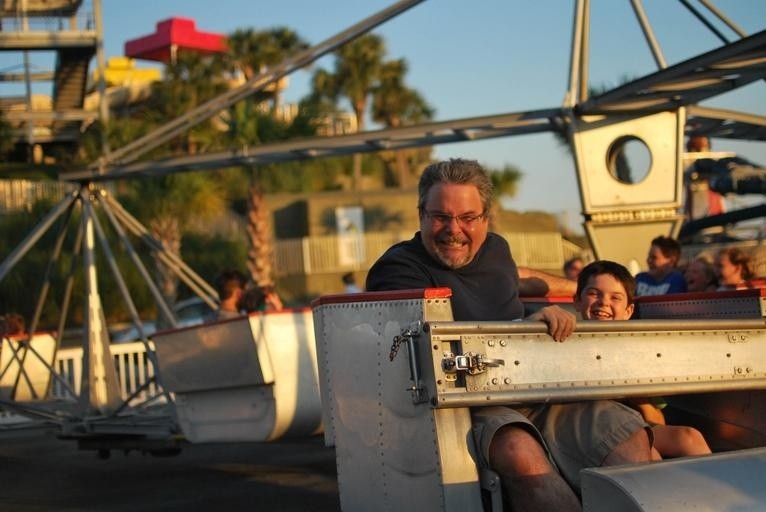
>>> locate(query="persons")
[564,257,584,281]
[215,269,249,321]
[365,157,655,512]
[715,246,756,291]
[244,284,283,311]
[343,271,364,293]
[525,259,712,461]
[635,235,687,296]
[684,252,718,292]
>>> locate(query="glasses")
[420,205,488,225]
